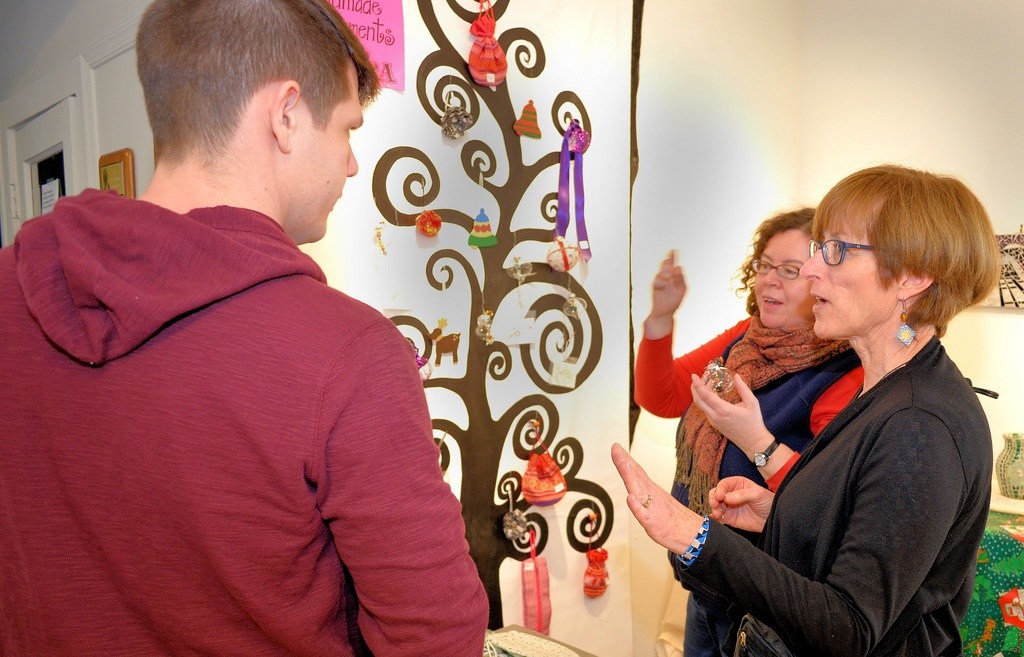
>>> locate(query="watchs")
[751,441,779,467]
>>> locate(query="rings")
[643,495,652,508]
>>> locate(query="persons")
[633,207,865,657]
[0,0,490,657]
[611,165,1001,657]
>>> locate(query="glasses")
[751,260,799,280]
[808,239,876,266]
